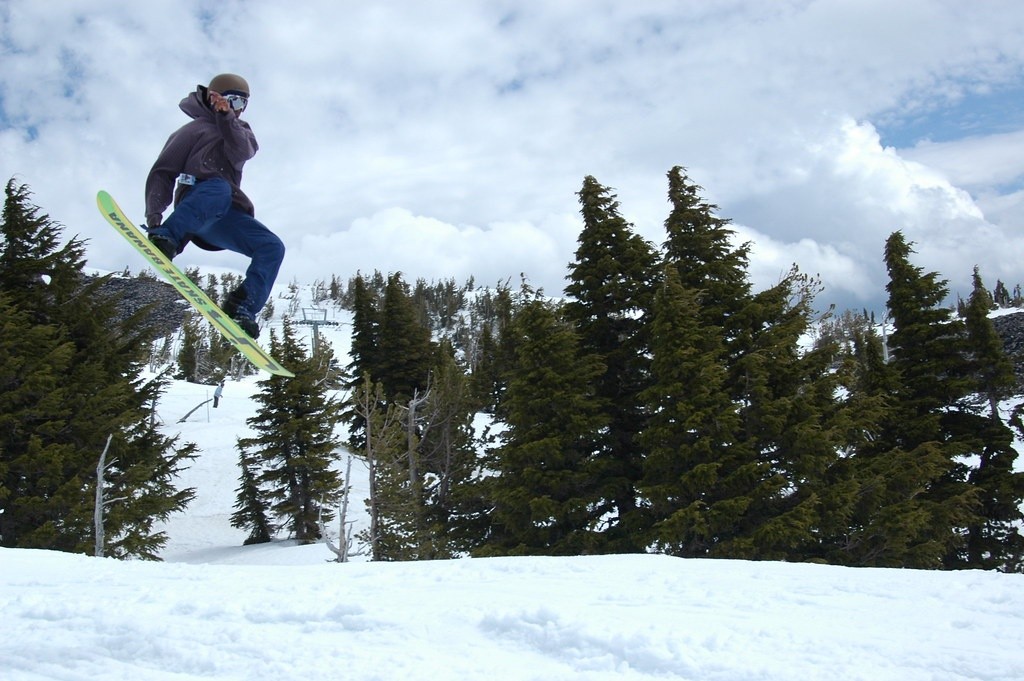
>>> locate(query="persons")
[139,73,285,341]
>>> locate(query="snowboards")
[93,188,297,380]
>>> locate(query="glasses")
[222,94,248,112]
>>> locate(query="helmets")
[206,74,250,100]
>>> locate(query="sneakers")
[150,234,176,262]
[228,314,259,339]
